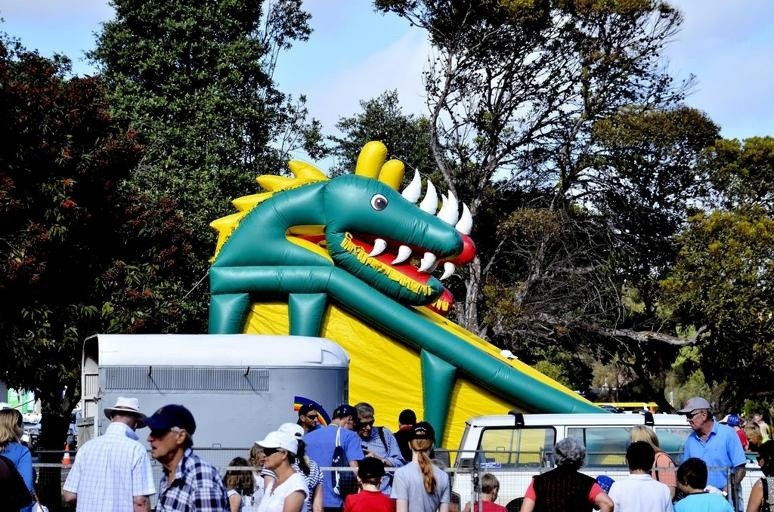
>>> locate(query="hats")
[399,409,416,425]
[104,396,148,429]
[501,349,519,360]
[279,423,305,441]
[332,405,362,422]
[677,396,711,415]
[298,403,316,415]
[719,414,732,423]
[139,404,196,435]
[359,457,386,479]
[407,421,436,444]
[254,431,299,455]
[727,415,739,426]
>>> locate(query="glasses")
[686,412,703,419]
[307,415,319,420]
[756,456,763,462]
[263,448,282,455]
[358,420,375,427]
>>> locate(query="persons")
[678,397,746,512]
[0,456,49,512]
[609,441,674,511]
[354,402,406,495]
[303,405,364,512]
[743,422,761,450]
[520,438,614,512]
[393,408,435,462]
[388,422,450,512]
[249,444,269,495]
[673,457,734,512]
[62,396,156,511]
[752,406,772,439]
[631,425,677,502]
[726,416,747,446]
[296,403,319,433]
[0,408,33,512]
[140,404,231,512]
[223,457,254,512]
[343,457,395,512]
[470,473,508,512]
[254,431,309,512]
[747,439,774,512]
[278,423,323,512]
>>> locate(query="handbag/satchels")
[21,488,49,512]
[656,454,689,504]
[331,426,358,500]
[760,477,771,512]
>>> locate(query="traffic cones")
[61,443,71,466]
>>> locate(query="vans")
[450,408,768,512]
